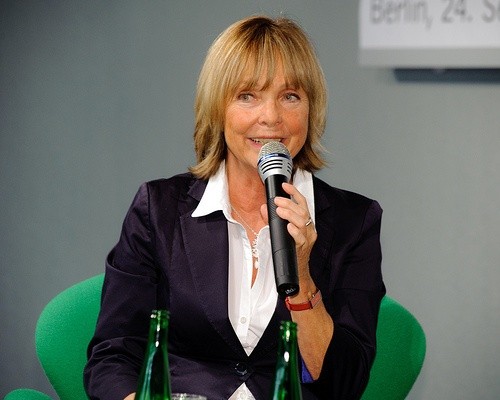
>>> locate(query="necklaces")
[230,203,258,257]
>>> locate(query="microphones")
[257,141,300,297]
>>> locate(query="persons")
[83,15,386,400]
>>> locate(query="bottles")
[272,320,304,400]
[134,309,172,400]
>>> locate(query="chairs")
[5,273,427,400]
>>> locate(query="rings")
[306,217,313,226]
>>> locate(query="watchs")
[285,287,322,311]
